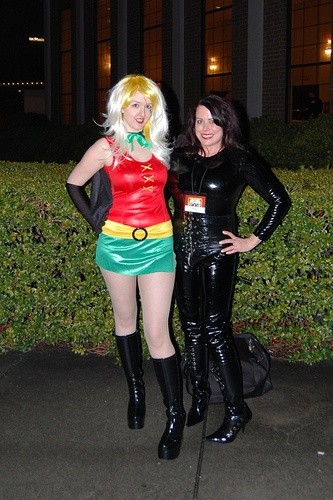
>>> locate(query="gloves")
[66,183,109,233]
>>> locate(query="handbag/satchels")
[180,332,272,402]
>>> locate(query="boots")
[186,344,209,427]
[151,352,186,459]
[203,360,252,443]
[114,330,145,429]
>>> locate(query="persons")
[164,93,289,444]
[67,75,187,460]
